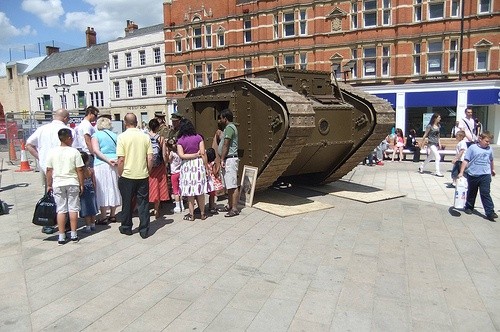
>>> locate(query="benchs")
[385,137,459,161]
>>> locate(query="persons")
[144,119,171,219]
[116,113,154,239]
[358,128,405,166]
[458,107,483,148]
[26,105,99,234]
[451,121,460,138]
[194,109,241,217]
[91,117,122,225]
[457,131,498,222]
[418,112,445,177]
[46,128,85,246]
[403,128,421,162]
[154,113,189,213]
[176,119,208,221]
[446,131,468,188]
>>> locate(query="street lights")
[53,84,70,110]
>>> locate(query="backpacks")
[146,133,163,168]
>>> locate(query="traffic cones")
[15,140,33,172]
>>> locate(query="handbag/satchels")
[32,196,58,226]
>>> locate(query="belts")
[225,156,237,158]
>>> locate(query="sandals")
[108,217,116,222]
[182,205,239,221]
[95,218,107,225]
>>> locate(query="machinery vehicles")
[175,67,395,188]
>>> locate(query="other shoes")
[171,209,180,213]
[464,207,472,214]
[487,213,498,219]
[70,236,79,242]
[119,226,132,235]
[81,227,96,233]
[58,240,65,245]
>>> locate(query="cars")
[0,102,18,144]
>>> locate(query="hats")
[170,113,186,121]
